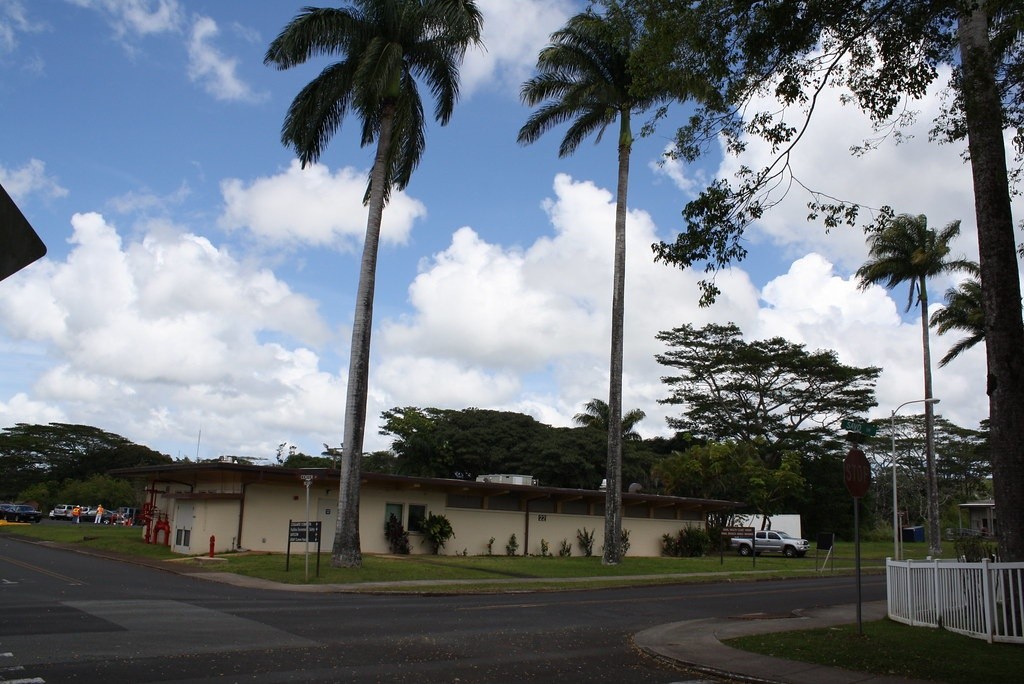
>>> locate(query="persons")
[94,504,104,524]
[72,505,80,524]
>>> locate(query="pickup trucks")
[731,530,810,558]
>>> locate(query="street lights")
[892,399,940,561]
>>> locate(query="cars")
[80,506,123,525]
[49,510,55,520]
[0,504,42,523]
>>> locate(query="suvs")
[53,504,76,521]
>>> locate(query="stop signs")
[844,449,872,497]
